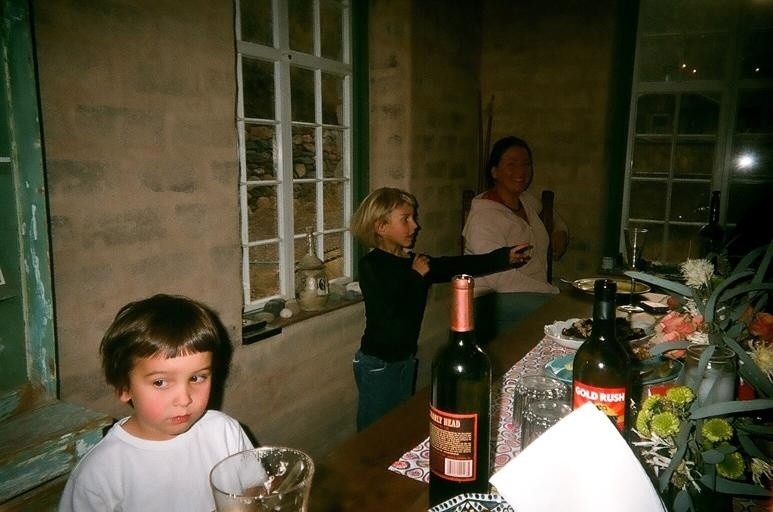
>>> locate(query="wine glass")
[617,227,648,314]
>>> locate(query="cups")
[514,377,571,454]
[209,447,316,512]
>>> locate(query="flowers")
[630,258,773,493]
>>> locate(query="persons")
[460,136,569,331]
[345,187,529,431]
[56,292,268,511]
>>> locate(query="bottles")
[428,274,492,508]
[572,278,630,437]
[674,344,737,419]
[696,189,730,277]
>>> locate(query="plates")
[543,354,684,390]
[548,318,656,350]
[572,278,651,297]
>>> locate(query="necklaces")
[495,190,522,212]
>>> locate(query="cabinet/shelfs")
[619,0,773,268]
